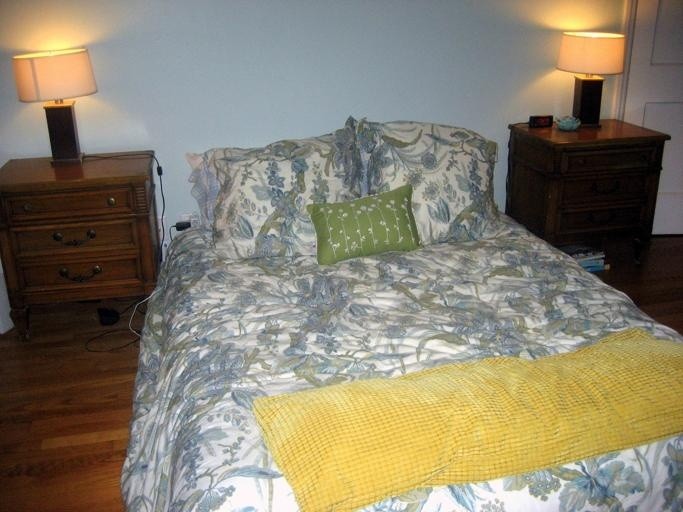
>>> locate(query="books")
[558,243,611,274]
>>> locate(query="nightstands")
[508,116,670,272]
[0,151,167,343]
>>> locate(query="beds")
[144,208,683,511]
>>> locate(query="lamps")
[11,44,100,168]
[557,29,627,127]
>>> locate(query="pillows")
[306,184,420,265]
[204,127,357,265]
[361,119,498,245]
[187,147,248,247]
[345,116,370,197]
